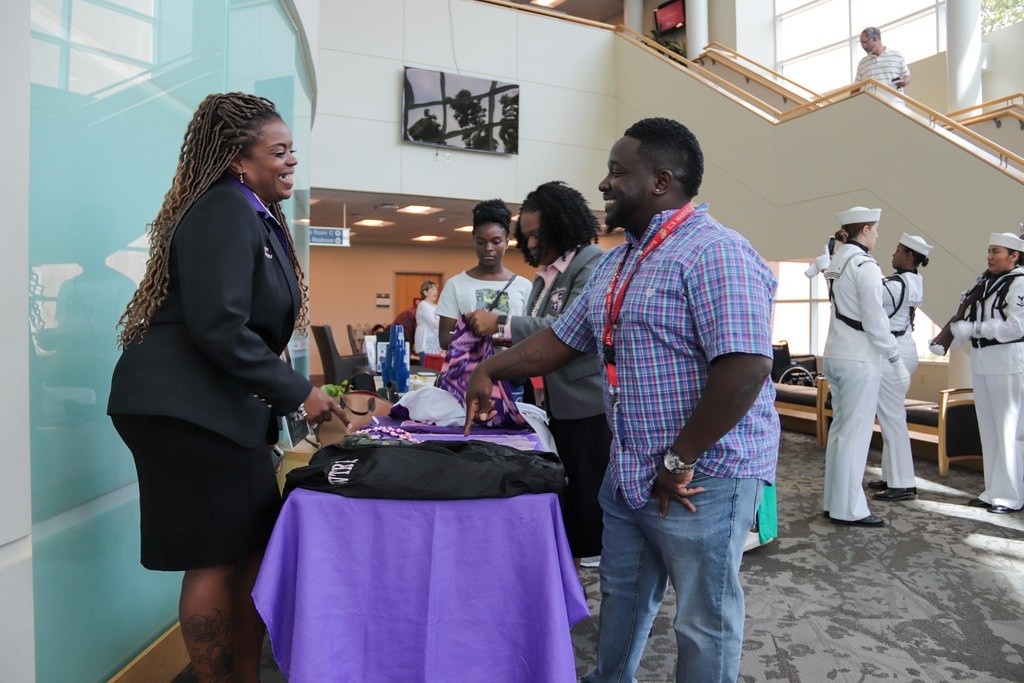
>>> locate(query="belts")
[891,325,909,337]
[970,334,1024,349]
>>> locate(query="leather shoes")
[823,510,884,527]
[968,499,992,508]
[872,487,918,501]
[987,505,1024,514]
[868,480,887,490]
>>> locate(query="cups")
[381,325,409,393]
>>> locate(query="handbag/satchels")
[301,370,395,449]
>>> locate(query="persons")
[434,198,534,402]
[361,280,446,363]
[850,27,911,107]
[815,206,933,527]
[465,119,779,682]
[466,180,614,602]
[950,233,1024,514]
[105,90,349,683]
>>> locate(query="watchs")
[496,315,507,338]
[663,447,697,474]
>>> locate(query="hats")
[899,232,933,257]
[989,232,1024,252]
[835,206,881,225]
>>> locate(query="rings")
[312,423,318,430]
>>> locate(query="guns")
[931,228,1024,356]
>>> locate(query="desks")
[254,399,590,683]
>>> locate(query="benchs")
[770,377,822,447]
[820,371,985,476]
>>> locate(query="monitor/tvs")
[654,0,685,37]
[402,67,520,154]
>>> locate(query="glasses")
[860,38,871,45]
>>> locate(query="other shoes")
[581,555,601,567]
[742,530,773,553]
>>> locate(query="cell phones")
[271,446,284,468]
[828,236,836,255]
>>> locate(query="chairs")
[310,324,368,385]
[347,324,365,355]
